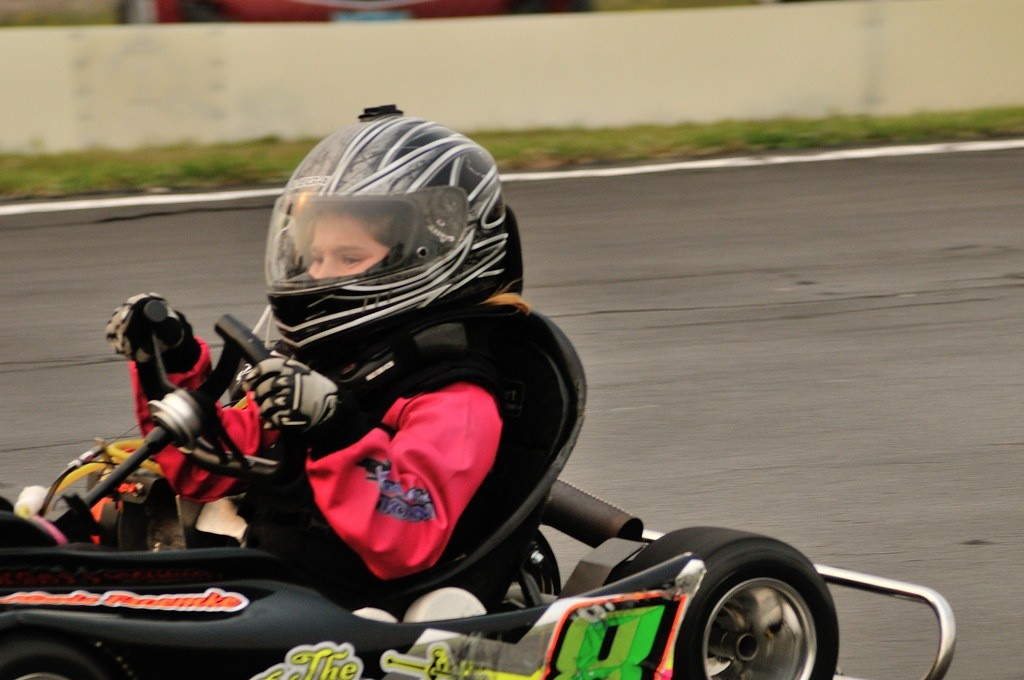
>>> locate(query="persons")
[105,117,509,582]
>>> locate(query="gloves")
[105,291,185,364]
[242,359,338,434]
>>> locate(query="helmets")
[264,114,509,368]
